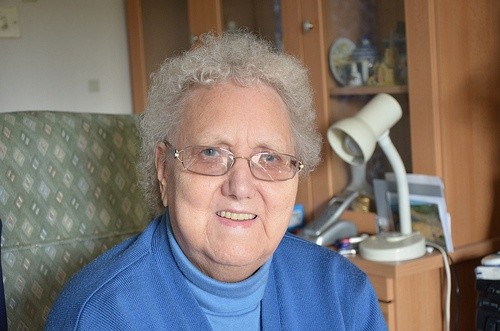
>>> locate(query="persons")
[45,30,388,331]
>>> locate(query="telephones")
[296,192,359,247]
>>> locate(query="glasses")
[162,139,305,182]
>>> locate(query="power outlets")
[0,4,21,39]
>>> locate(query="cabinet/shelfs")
[125,0,500,331]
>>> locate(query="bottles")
[352,35,379,86]
[391,22,408,85]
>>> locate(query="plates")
[329,37,357,86]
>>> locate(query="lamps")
[326,92,429,262]
